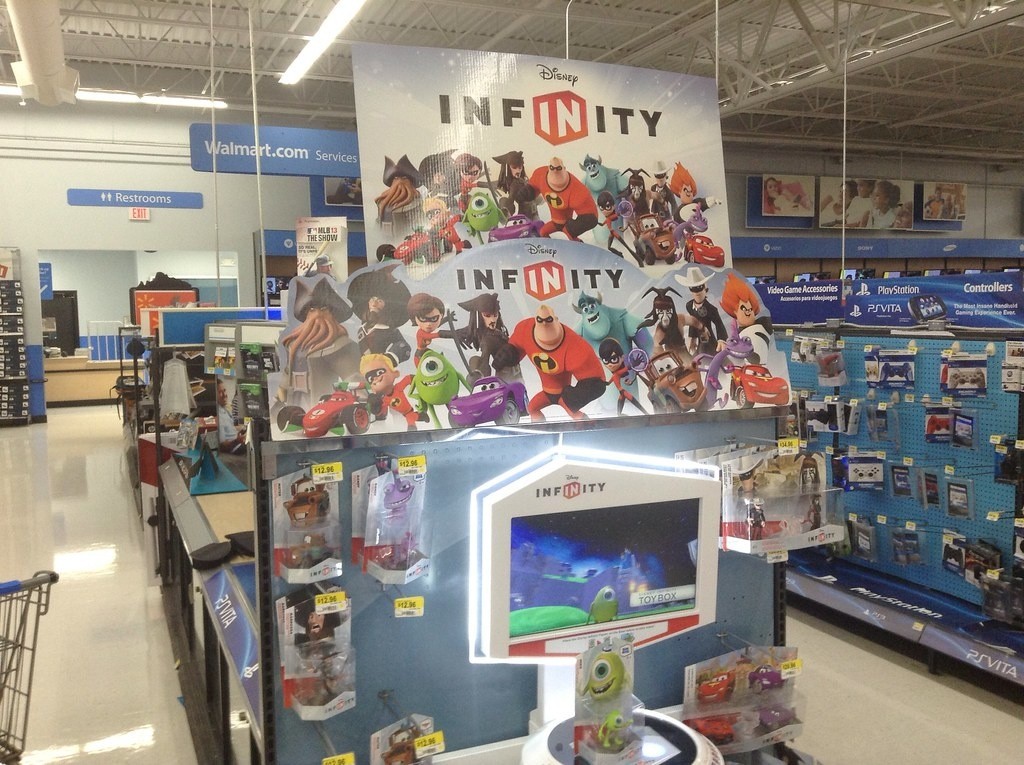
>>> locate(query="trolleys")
[1,571,59,765]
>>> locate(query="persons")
[746,497,767,541]
[766,177,814,215]
[305,255,338,280]
[349,183,362,205]
[820,178,913,229]
[923,186,967,220]
[807,494,822,529]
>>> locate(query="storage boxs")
[843,404,863,435]
[964,539,1003,591]
[939,352,987,398]
[924,404,960,446]
[806,400,846,432]
[865,350,915,390]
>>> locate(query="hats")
[316,255,334,267]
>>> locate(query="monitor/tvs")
[509,497,700,639]
